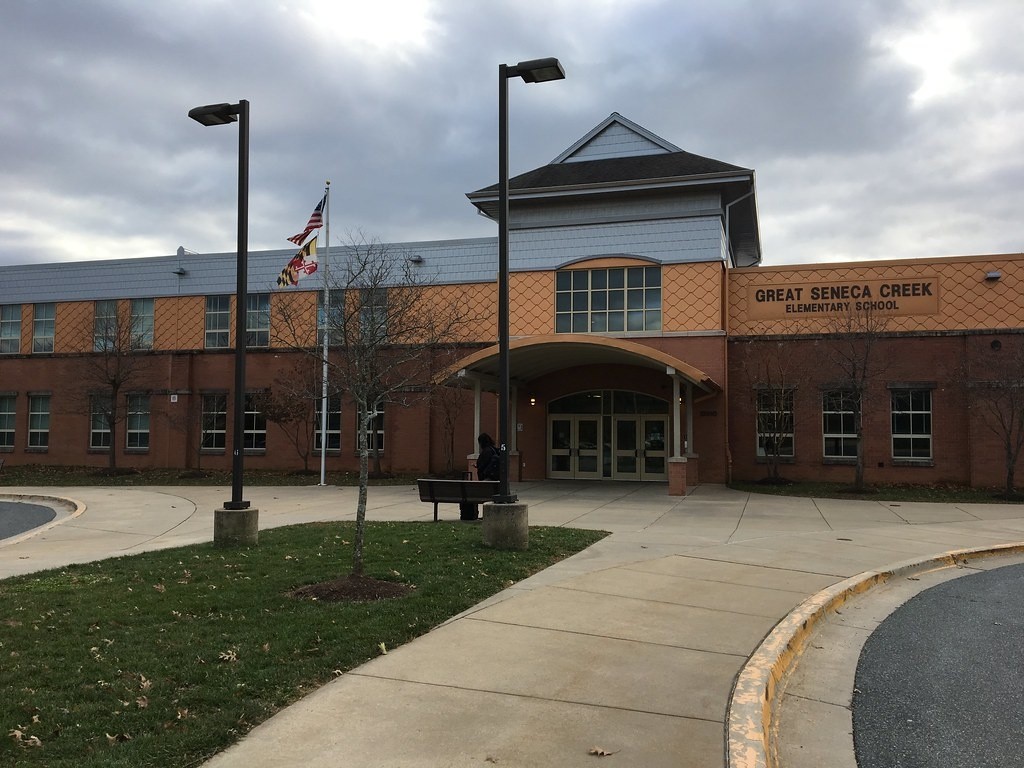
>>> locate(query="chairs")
[416,478,511,522]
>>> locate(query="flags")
[286,194,327,246]
[276,235,319,289]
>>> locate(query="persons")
[471,432,502,521]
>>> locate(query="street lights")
[481,56,566,550]
[187,99,260,544]
